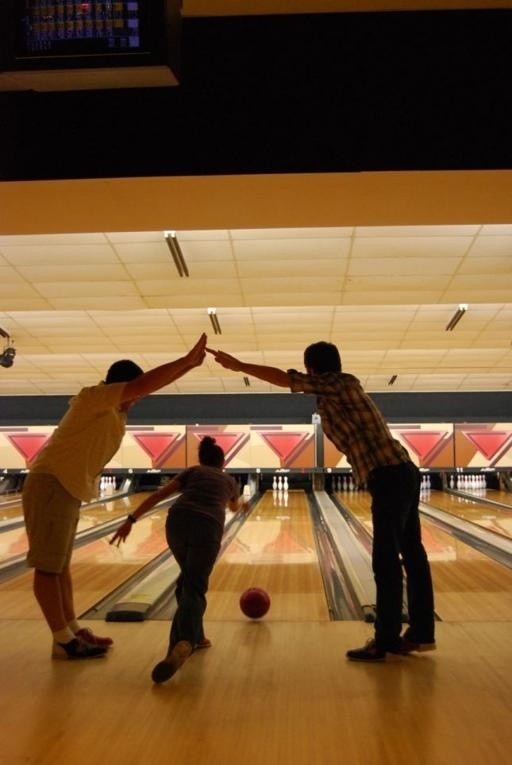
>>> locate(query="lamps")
[0,348,16,368]
[243,376,250,386]
[387,375,397,386]
[208,308,222,335]
[164,231,189,277]
[445,303,467,332]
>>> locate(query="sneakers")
[398,633,437,653]
[345,639,401,662]
[52,637,107,660]
[151,638,213,685]
[75,628,112,647]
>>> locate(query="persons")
[20,331,209,662]
[107,431,252,684]
[202,340,438,663]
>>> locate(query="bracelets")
[129,515,139,526]
